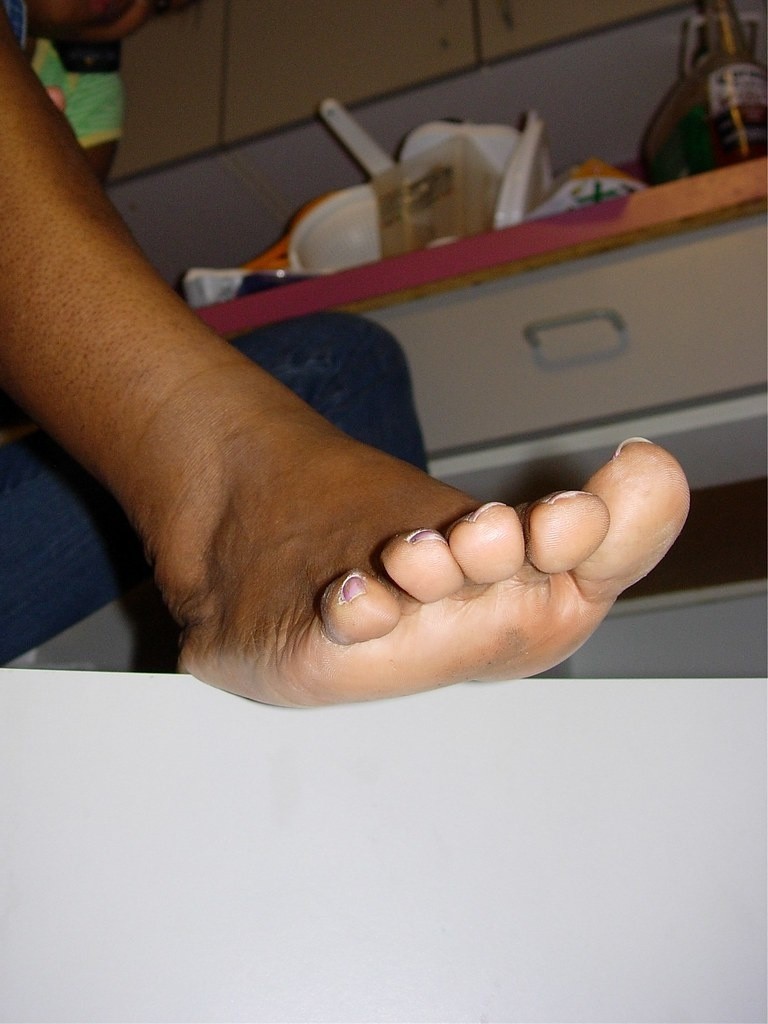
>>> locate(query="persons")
[2,1,692,710]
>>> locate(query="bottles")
[694,1,768,167]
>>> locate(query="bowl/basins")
[372,135,502,257]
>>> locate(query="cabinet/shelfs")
[109,0,767,514]
